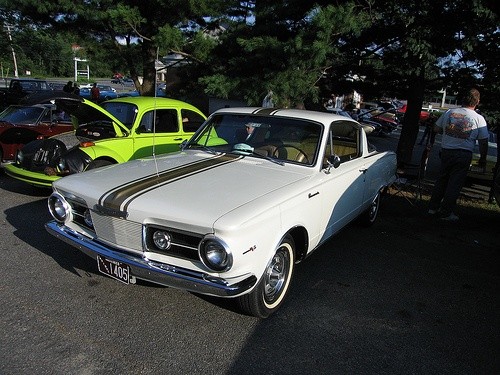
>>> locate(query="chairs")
[390,145,431,209]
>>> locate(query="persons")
[63,81,80,95]
[90,83,100,104]
[243,122,256,140]
[427,85,489,221]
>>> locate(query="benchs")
[261,136,357,162]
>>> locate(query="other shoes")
[428,207,441,214]
[441,213,459,221]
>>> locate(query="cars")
[335,98,431,135]
[43,106,395,319]
[0,78,229,190]
